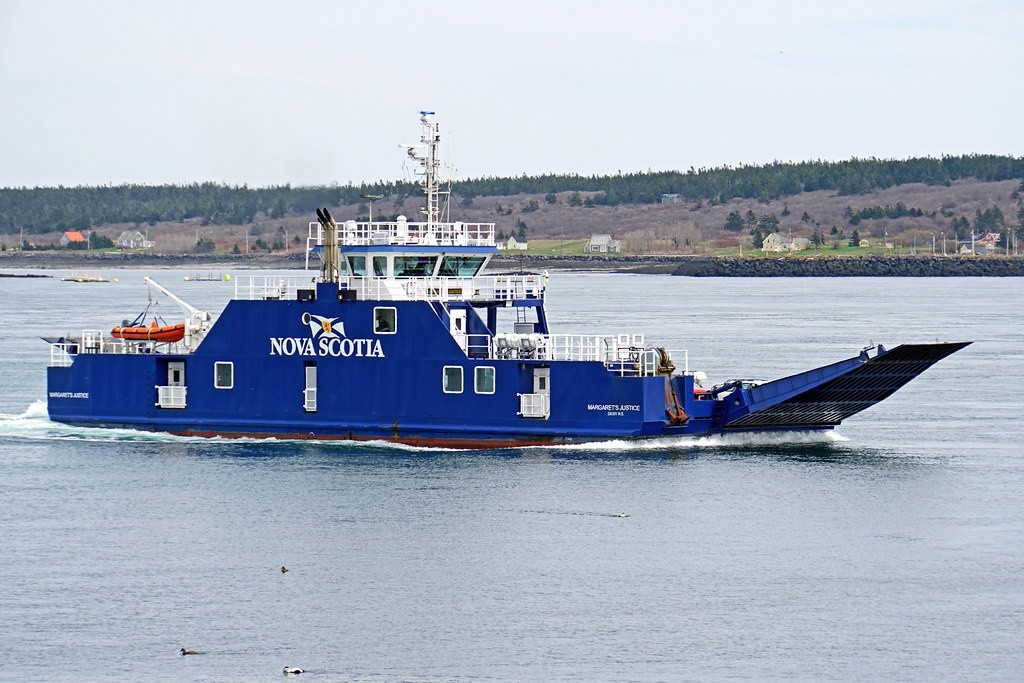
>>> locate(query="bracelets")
[381,329,384,331]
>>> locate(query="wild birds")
[281,565,289,573]
[180,648,201,655]
[283,665,305,674]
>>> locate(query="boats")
[108,322,185,344]
[36,105,975,456]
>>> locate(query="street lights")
[941,232,946,254]
[930,233,935,254]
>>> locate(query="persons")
[376,315,391,332]
